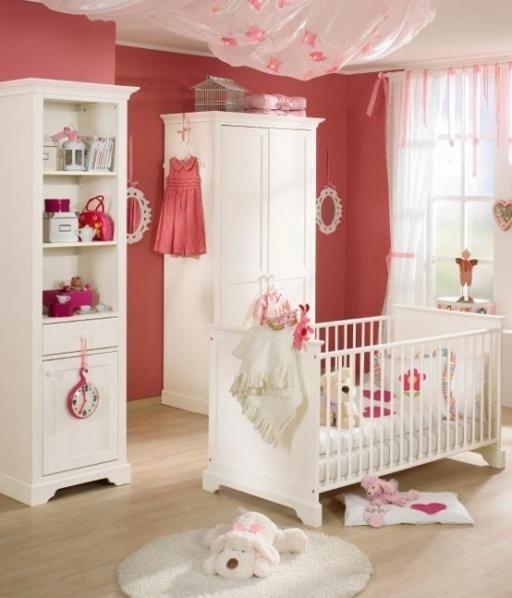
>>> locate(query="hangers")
[162,140,204,167]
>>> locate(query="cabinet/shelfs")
[159,109,325,416]
[0,76,138,508]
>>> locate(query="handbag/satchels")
[78,195,113,242]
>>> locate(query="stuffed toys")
[318,365,363,427]
[201,510,308,583]
[293,304,316,349]
[354,472,420,508]
[64,275,85,291]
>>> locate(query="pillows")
[374,346,456,423]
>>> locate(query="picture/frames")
[493,199,512,231]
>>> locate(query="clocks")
[67,367,99,419]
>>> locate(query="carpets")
[120,529,374,594]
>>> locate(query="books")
[82,130,116,172]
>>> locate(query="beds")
[203,308,501,524]
